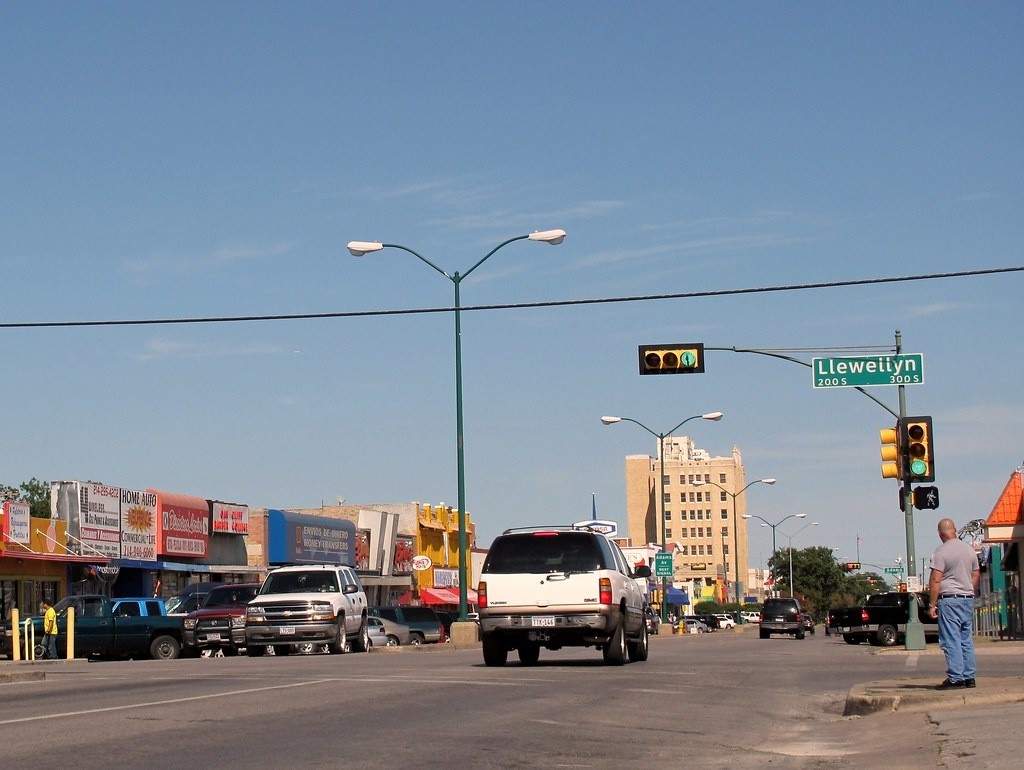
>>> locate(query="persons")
[927,519,980,690]
[648,613,686,636]
[41,599,59,659]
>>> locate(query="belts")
[938,595,975,599]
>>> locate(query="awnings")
[0,549,267,574]
[666,587,690,605]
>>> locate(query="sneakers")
[965,678,976,688]
[936,677,966,690]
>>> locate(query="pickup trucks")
[1,595,186,662]
[827,592,940,645]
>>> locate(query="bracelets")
[928,603,935,608]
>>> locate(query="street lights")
[602,412,722,621]
[349,228,568,620]
[691,479,819,623]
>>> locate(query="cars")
[824,613,840,636]
[804,616,814,635]
[301,617,412,654]
[645,606,761,632]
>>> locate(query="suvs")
[478,520,650,666]
[758,596,805,639]
[371,606,446,645]
[167,563,369,655]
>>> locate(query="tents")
[393,587,478,613]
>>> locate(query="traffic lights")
[869,580,877,584]
[638,345,704,375]
[880,426,902,479]
[915,488,939,510]
[900,582,907,590]
[848,564,859,568]
[900,417,934,482]
